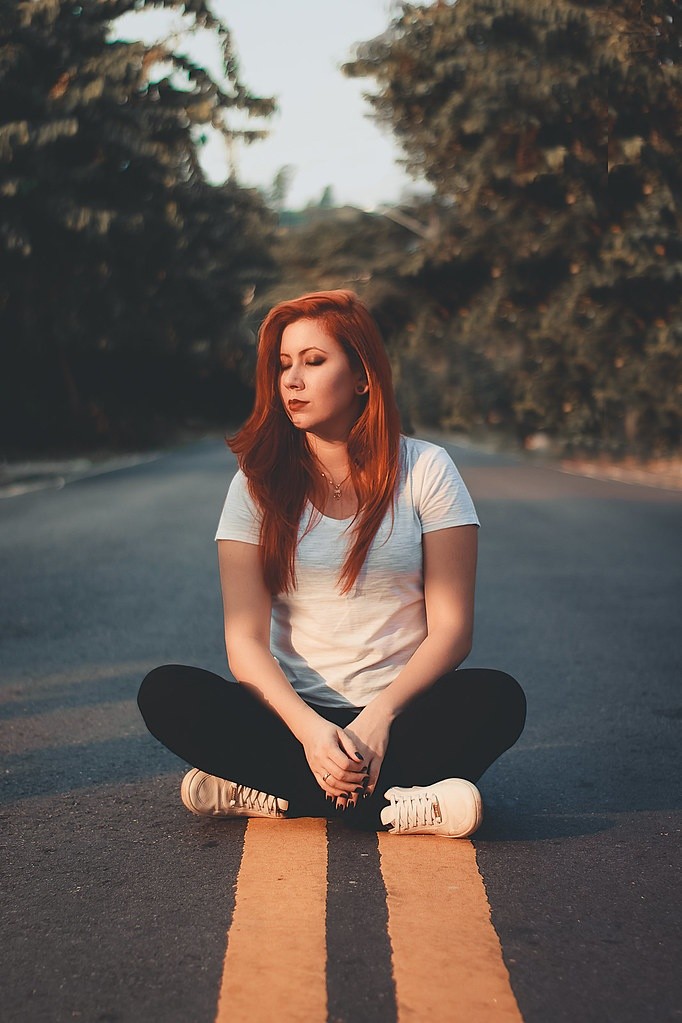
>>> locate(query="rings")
[323,774,330,781]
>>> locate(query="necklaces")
[322,471,352,500]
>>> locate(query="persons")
[139,291,528,838]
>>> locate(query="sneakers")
[180,767,289,820]
[381,775,483,837]
